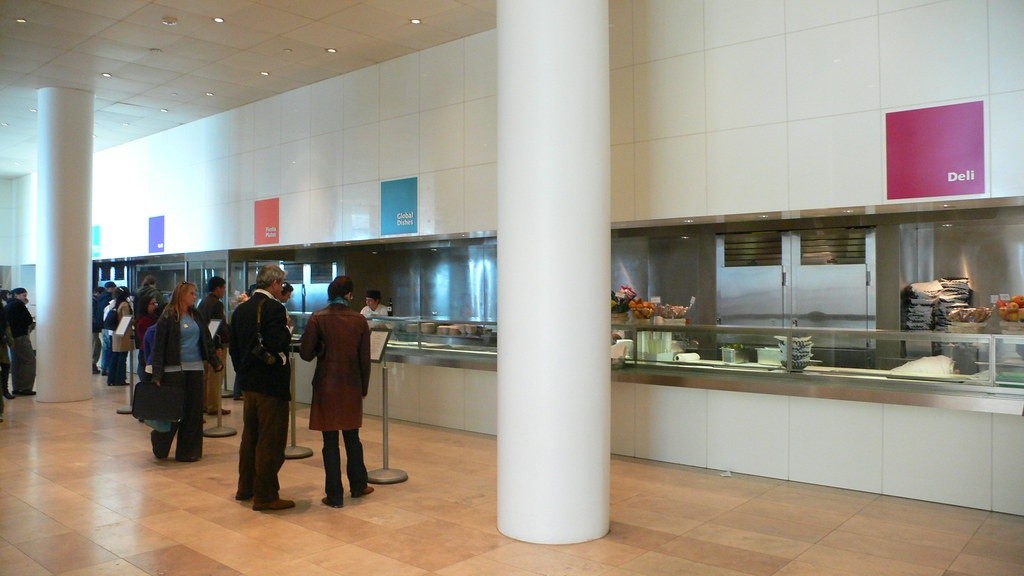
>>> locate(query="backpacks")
[103,302,122,331]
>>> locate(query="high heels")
[350,487,374,497]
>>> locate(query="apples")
[994,295,1024,322]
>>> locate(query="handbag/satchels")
[131,381,187,423]
[316,341,326,358]
[236,343,291,396]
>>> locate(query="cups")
[631,308,656,319]
[655,306,688,318]
[466,325,477,333]
[617,340,634,360]
[636,331,672,360]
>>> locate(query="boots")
[0,364,15,399]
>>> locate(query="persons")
[91,273,230,462]
[298,275,375,507]
[360,289,389,318]
[279,281,294,304]
[233,284,257,400]
[228,264,296,512]
[0,283,36,423]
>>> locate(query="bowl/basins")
[959,308,992,322]
[779,339,812,371]
[998,308,1024,321]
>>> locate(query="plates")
[406,324,417,332]
[907,277,970,330]
[421,323,459,335]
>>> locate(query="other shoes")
[253,499,295,511]
[102,371,108,376]
[322,497,344,508]
[236,492,253,501]
[203,407,209,413]
[92,363,100,374]
[12,390,22,395]
[108,383,115,386]
[116,381,129,385]
[233,395,243,400]
[21,390,36,395]
[208,409,231,415]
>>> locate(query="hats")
[366,290,382,297]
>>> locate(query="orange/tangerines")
[628,300,655,320]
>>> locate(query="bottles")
[387,298,393,315]
